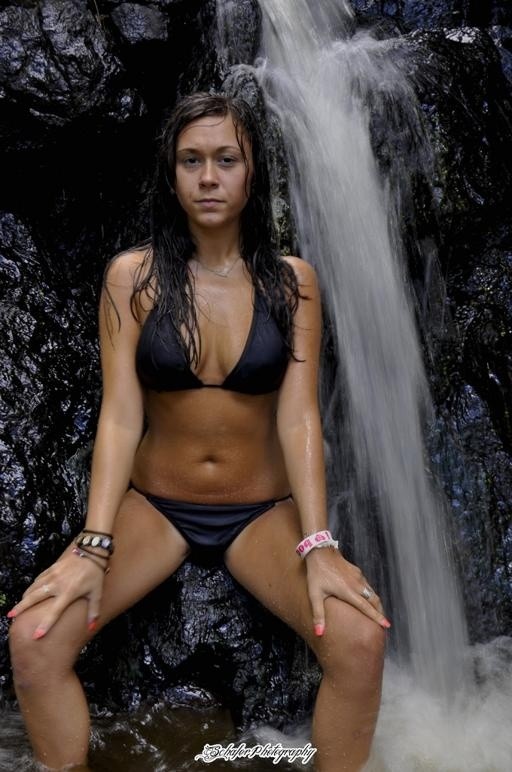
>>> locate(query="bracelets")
[6,91,391,772]
[66,528,117,572]
[294,531,339,561]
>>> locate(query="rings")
[360,584,375,601]
